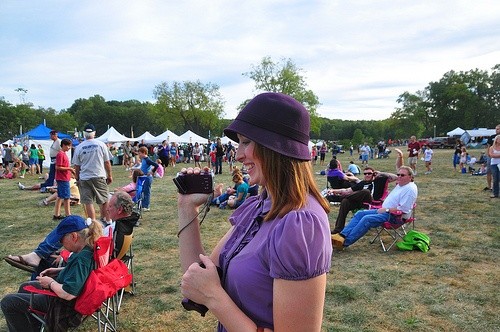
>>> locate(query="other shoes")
[482,186,492,191]
[37,198,49,206]
[331,233,345,250]
[53,214,66,220]
[489,193,499,198]
[331,229,341,234]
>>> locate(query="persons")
[332,167,399,234]
[0,215,103,332]
[407,136,421,175]
[452,124,500,198]
[43,177,81,206]
[108,168,144,203]
[105,141,237,178]
[53,139,76,219]
[421,143,434,175]
[0,142,45,179]
[327,156,342,171]
[138,147,159,211]
[18,172,49,190]
[45,130,62,186]
[4,191,135,281]
[327,159,361,183]
[208,163,259,210]
[343,161,361,176]
[331,148,418,253]
[311,137,410,165]
[71,123,112,227]
[178,92,333,332]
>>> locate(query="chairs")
[363,180,388,209]
[117,233,136,314]
[127,176,152,219]
[327,176,344,205]
[369,202,416,252]
[23,227,116,332]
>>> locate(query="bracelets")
[256,325,264,332]
[48,280,55,289]
[386,208,389,213]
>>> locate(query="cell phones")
[373,172,377,176]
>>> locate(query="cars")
[427,137,456,149]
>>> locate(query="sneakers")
[17,182,24,190]
[425,171,433,175]
[142,207,151,212]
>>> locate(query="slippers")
[4,255,38,273]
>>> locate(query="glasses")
[364,172,373,175]
[396,174,407,177]
[233,179,239,183]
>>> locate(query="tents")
[466,128,497,136]
[447,127,466,137]
[221,135,239,146]
[13,124,71,169]
[95,126,138,143]
[178,129,209,145]
[138,131,156,145]
[155,130,179,145]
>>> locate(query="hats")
[223,92,311,161]
[83,124,96,133]
[47,215,89,244]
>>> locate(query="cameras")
[173,168,216,195]
